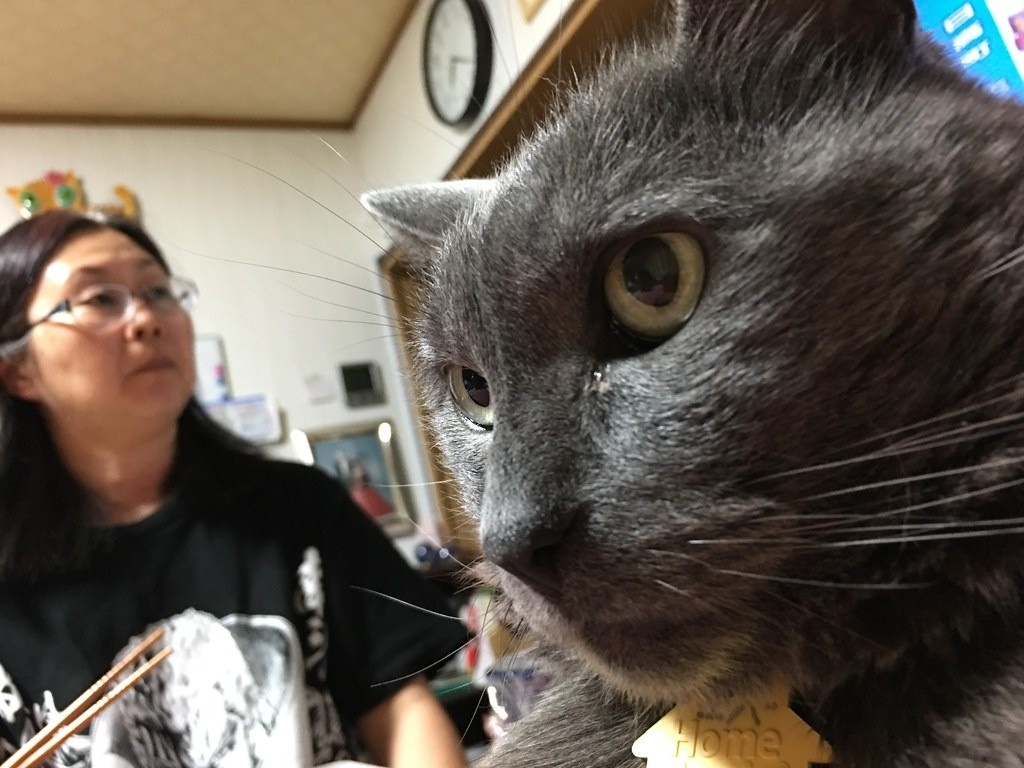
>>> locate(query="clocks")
[420,1,493,127]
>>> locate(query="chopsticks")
[0,627,175,768]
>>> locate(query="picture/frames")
[307,417,419,542]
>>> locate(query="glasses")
[0,274,200,357]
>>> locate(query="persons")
[332,450,394,518]
[0,210,477,768]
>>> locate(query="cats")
[359,1,1024,767]
